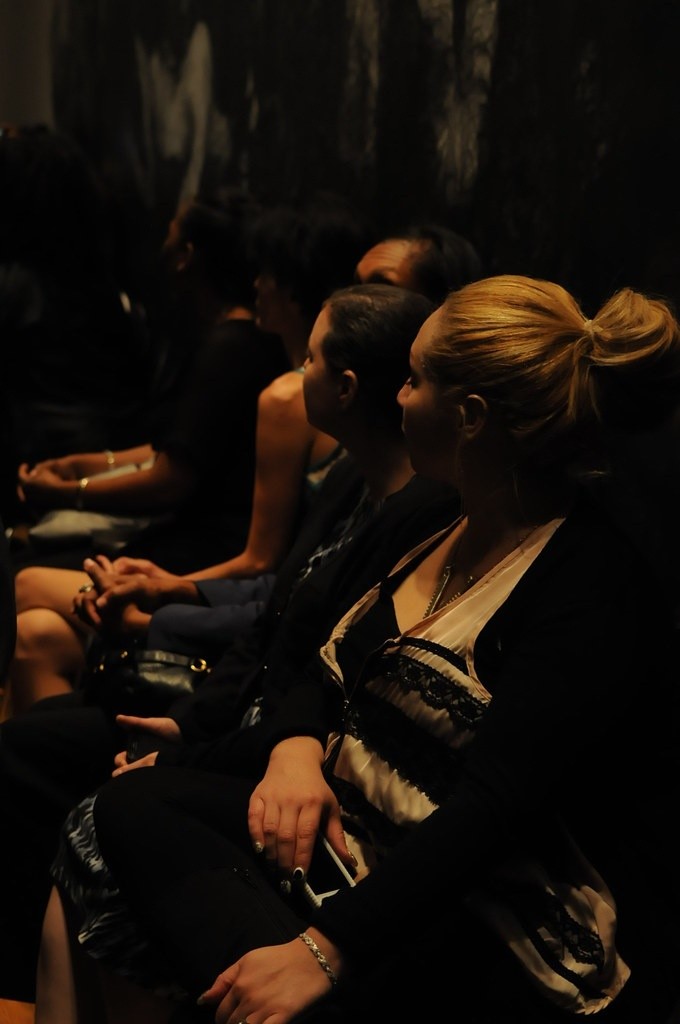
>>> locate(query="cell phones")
[299,829,356,912]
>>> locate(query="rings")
[79,585,92,593]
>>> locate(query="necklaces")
[422,526,536,618]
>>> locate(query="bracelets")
[299,933,338,986]
[77,477,88,494]
[104,450,115,471]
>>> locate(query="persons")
[0,1,680,1023]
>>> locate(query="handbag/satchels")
[92,651,213,718]
